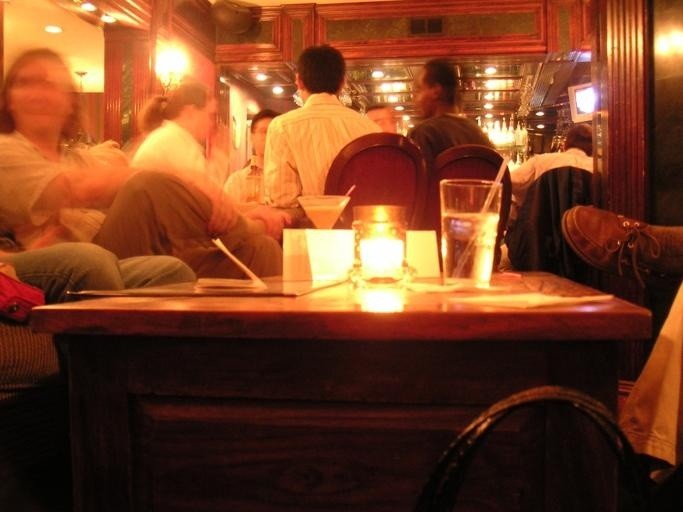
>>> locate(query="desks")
[30,272,654,511]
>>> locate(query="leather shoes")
[561,205,683,292]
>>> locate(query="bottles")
[501,119,529,147]
[557,137,566,153]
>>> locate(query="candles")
[362,292,405,314]
[358,238,405,272]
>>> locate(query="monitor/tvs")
[568,83,594,123]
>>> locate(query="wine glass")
[298,194,352,229]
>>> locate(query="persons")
[264,45,496,227]
[620,282,682,483]
[2,44,292,303]
[498,123,594,272]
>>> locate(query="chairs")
[421,145,511,272]
[504,165,591,284]
[322,132,428,263]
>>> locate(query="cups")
[349,204,408,290]
[439,178,503,288]
